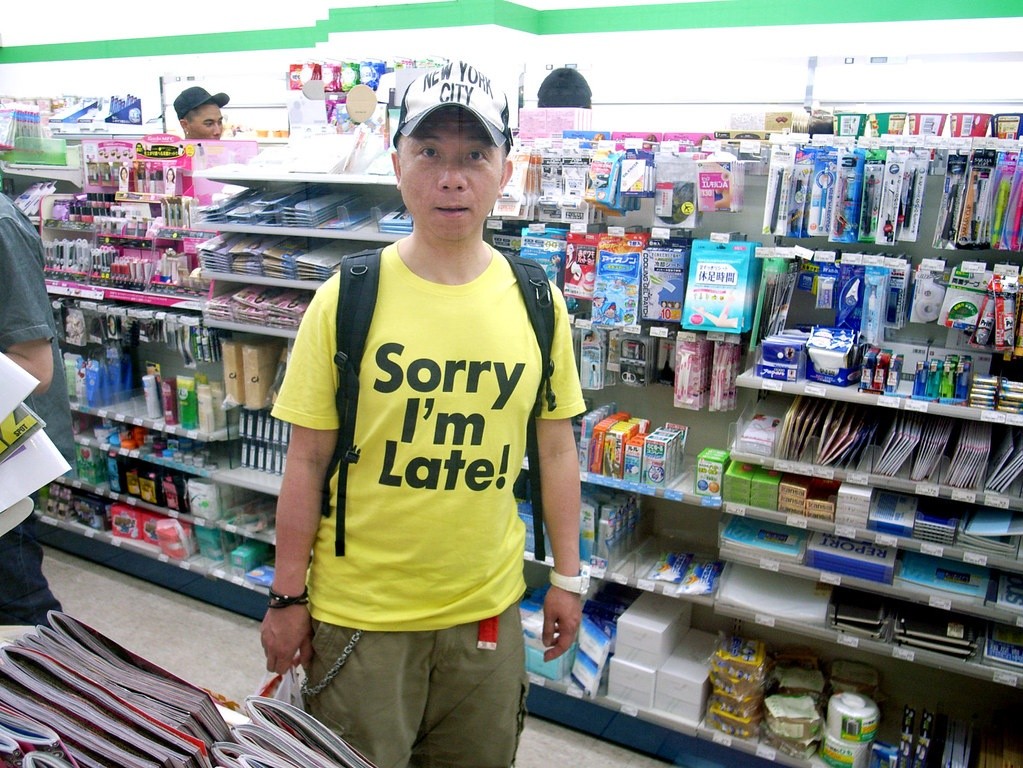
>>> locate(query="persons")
[0,191,77,627]
[173,86,230,141]
[537,67,593,110]
[261,60,590,768]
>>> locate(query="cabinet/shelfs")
[585,135,1023,768]
[522,141,771,768]
[22,189,240,615]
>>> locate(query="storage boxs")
[693,399,918,538]
[606,590,720,722]
[523,641,578,681]
[588,409,689,489]
[0,68,430,280]
[752,328,992,394]
[621,159,656,198]
[217,338,283,411]
[191,169,516,621]
[697,159,745,213]
[518,106,791,147]
[641,244,693,323]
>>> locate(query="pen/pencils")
[836,171,845,233]
[971,175,978,241]
[791,172,801,233]
[863,174,875,235]
[992,176,1007,245]
[770,169,784,234]
[903,167,916,228]
[887,180,894,237]
[941,175,959,248]
[818,168,828,232]
[1010,178,1023,249]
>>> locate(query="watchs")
[549,565,591,596]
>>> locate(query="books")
[720,507,1023,768]
[0,609,379,768]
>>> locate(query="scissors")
[656,340,674,385]
[622,366,637,382]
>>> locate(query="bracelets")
[267,585,309,609]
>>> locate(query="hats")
[170,81,234,119]
[380,57,521,149]
